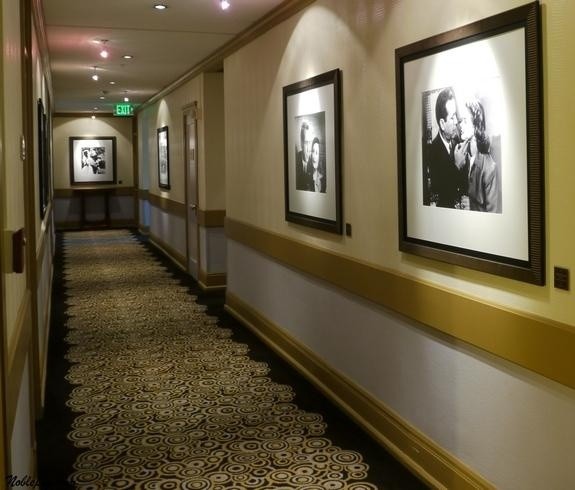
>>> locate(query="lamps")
[100,40,109,58]
[91,111,96,120]
[91,66,99,80]
[123,91,129,102]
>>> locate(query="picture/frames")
[157,126,169,190]
[393,1,545,288]
[69,137,118,184]
[38,97,50,219]
[283,68,343,236]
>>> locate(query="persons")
[425,88,469,206]
[295,122,312,191]
[81,150,97,175]
[306,137,326,193]
[87,148,98,174]
[458,100,499,213]
